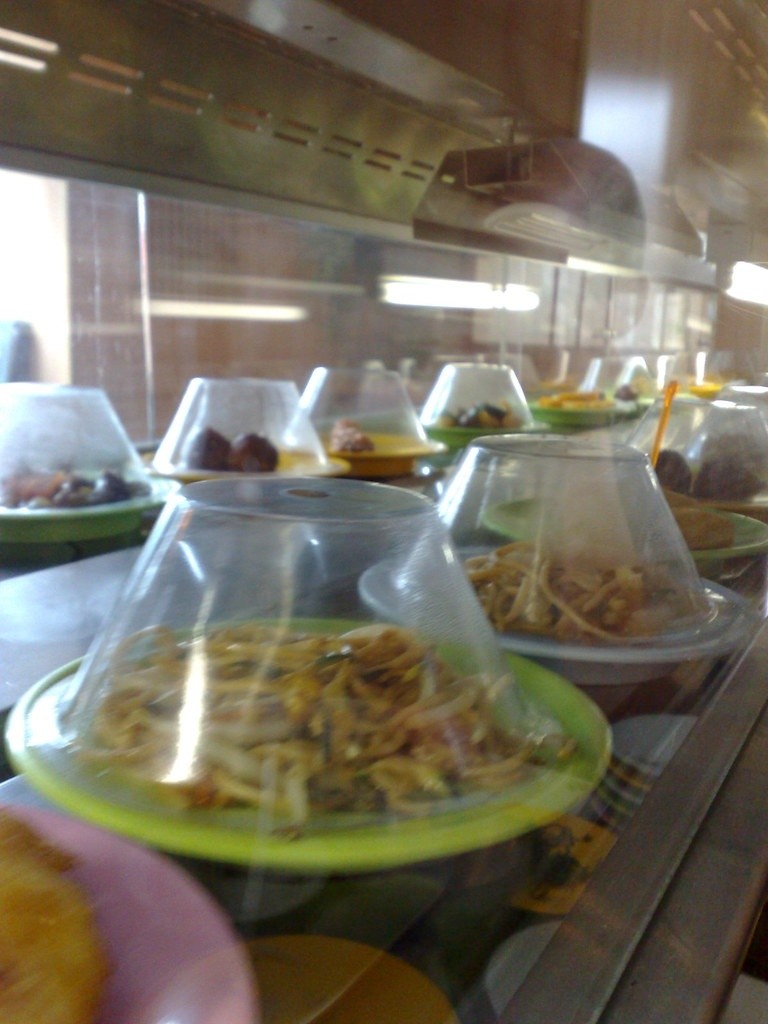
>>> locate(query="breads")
[662,490,733,548]
[0,807,106,1024]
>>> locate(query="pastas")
[452,538,708,635]
[86,622,578,826]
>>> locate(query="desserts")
[187,425,280,472]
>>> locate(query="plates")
[0,381,768,1024]
